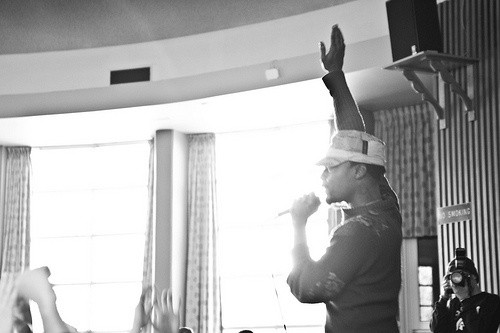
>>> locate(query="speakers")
[385,0,444,62]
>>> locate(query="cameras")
[449,248,472,287]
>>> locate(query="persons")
[429,248,500,333]
[17,265,77,333]
[286,23,403,333]
[131,284,182,333]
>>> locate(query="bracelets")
[438,294,449,301]
[459,296,470,302]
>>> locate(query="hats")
[444,257,479,282]
[317,131,386,167]
